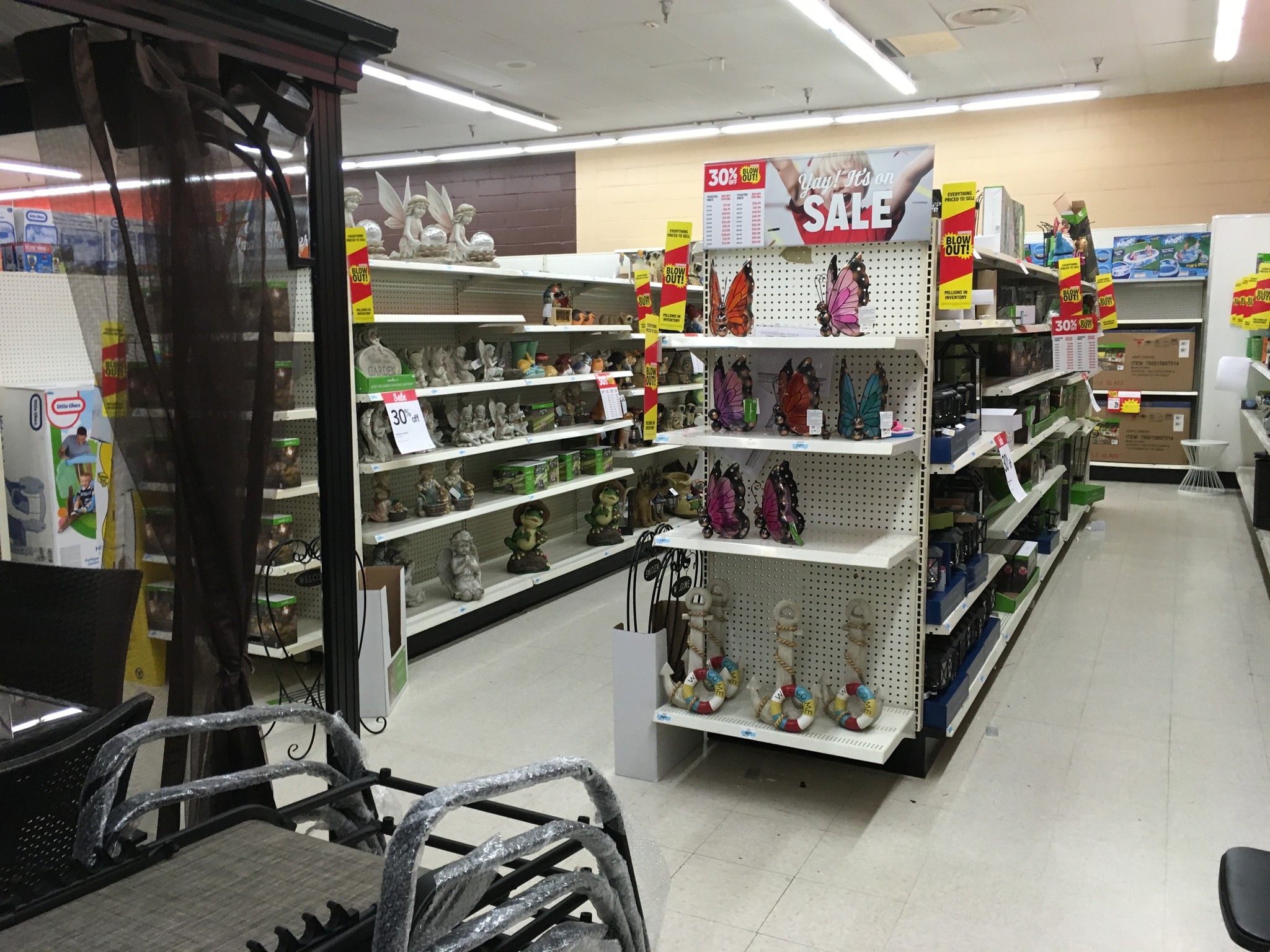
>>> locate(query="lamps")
[0,0,1246,203]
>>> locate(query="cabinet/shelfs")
[653,202,1102,779]
[1234,362,1270,570]
[1089,222,1211,471]
[131,256,706,661]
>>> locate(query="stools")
[1177,440,1230,497]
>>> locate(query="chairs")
[2,559,649,952]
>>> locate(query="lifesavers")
[682,669,729,715]
[770,684,814,734]
[705,657,739,698]
[833,684,875,731]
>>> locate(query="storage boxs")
[357,565,409,718]
[921,182,1210,731]
[610,598,706,783]
[354,357,415,395]
[494,443,614,496]
[0,195,300,650]
[518,400,555,433]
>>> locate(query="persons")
[767,143,935,244]
[58,473,96,533]
[345,171,529,607]
[58,425,93,483]
